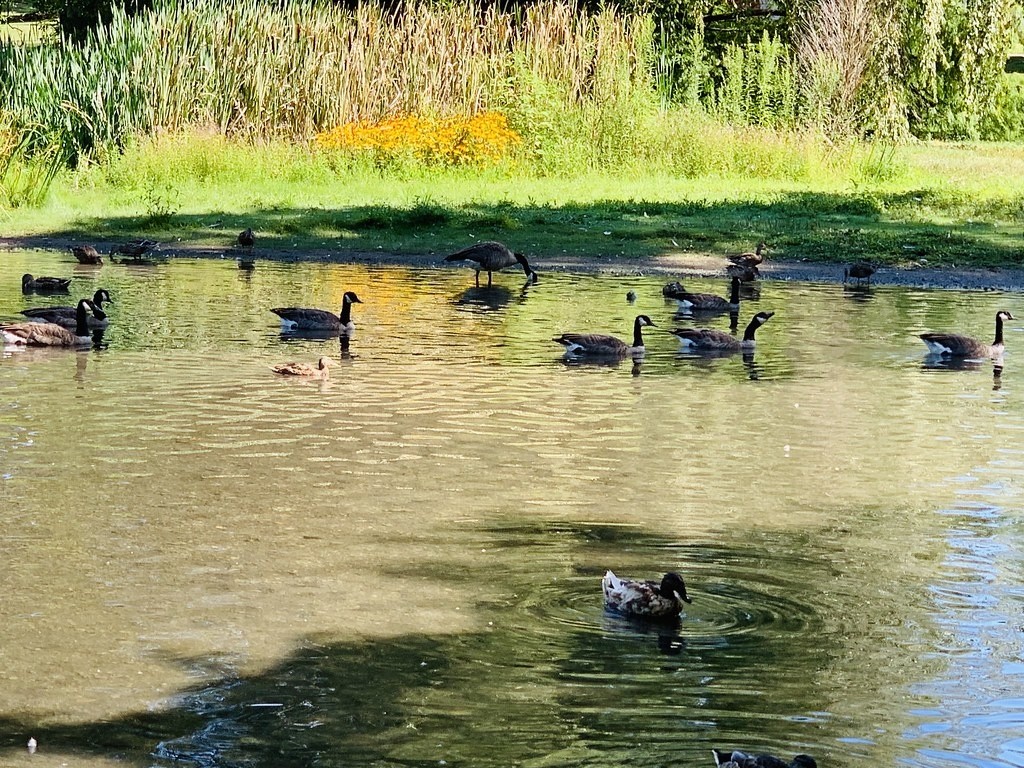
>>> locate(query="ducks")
[663,282,685,297]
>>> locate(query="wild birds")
[444,241,537,288]
[552,314,658,354]
[911,311,1018,359]
[22,274,72,296]
[267,356,341,375]
[602,569,691,617]
[727,243,770,267]
[109,238,161,261]
[727,263,761,281]
[238,228,256,248]
[270,292,364,330]
[70,245,104,264]
[844,262,877,286]
[0,290,115,346]
[668,311,774,349]
[674,275,741,310]
[712,749,817,768]
